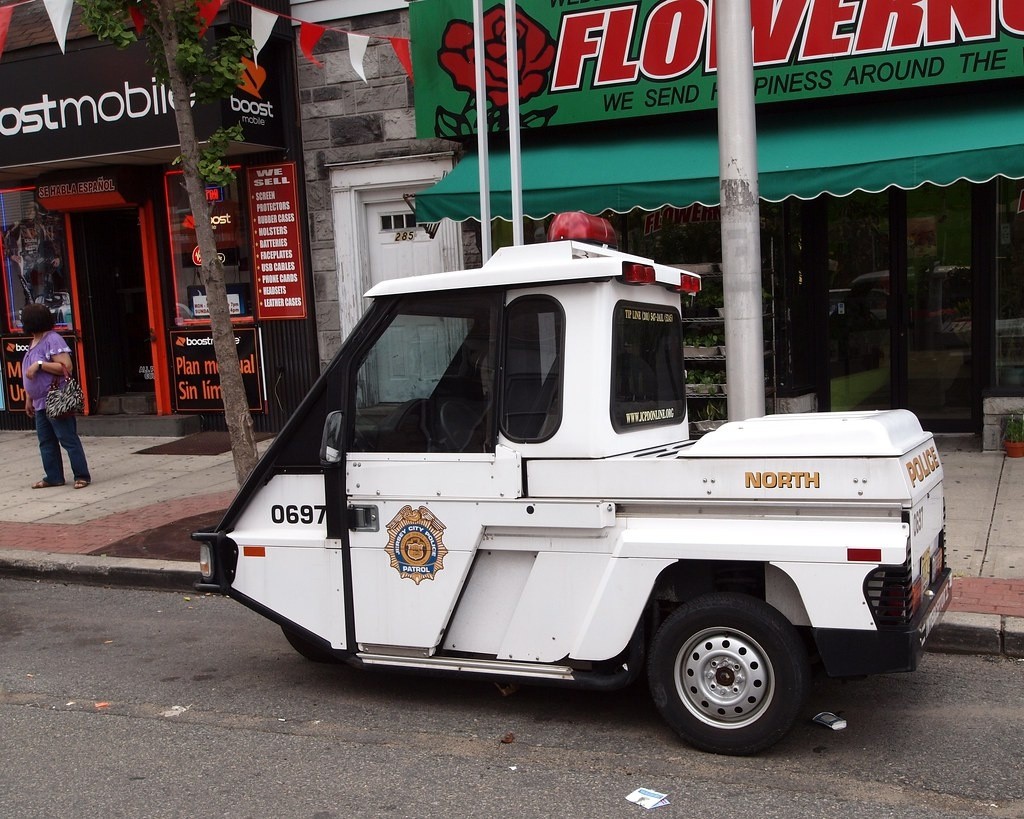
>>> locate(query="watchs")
[38,361,42,368]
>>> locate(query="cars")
[20,292,72,324]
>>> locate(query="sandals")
[74,480,88,489]
[32,479,65,489]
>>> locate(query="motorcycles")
[847,266,1024,344]
[191,212,953,757]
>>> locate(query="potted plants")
[715,295,724,317]
[688,401,728,430]
[685,370,727,394]
[1001,415,1024,458]
[683,336,726,357]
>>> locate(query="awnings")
[414,75,1024,224]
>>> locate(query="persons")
[21,302,92,489]
[4,200,62,306]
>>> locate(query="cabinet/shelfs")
[682,238,776,436]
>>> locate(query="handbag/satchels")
[45,362,85,418]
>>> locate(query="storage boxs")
[983,397,1024,450]
